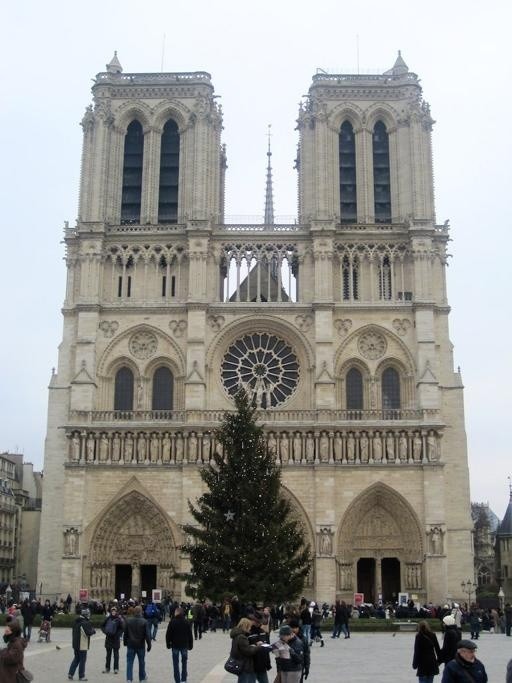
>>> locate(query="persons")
[413,616,488,683]
[322,598,511,639]
[431,527,443,555]
[319,529,332,554]
[1,594,325,683]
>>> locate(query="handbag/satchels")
[224,658,243,675]
[16,669,34,682]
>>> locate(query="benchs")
[391,621,419,638]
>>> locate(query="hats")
[249,610,266,623]
[280,626,292,635]
[456,640,477,649]
[443,616,456,626]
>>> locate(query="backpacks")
[106,619,117,634]
